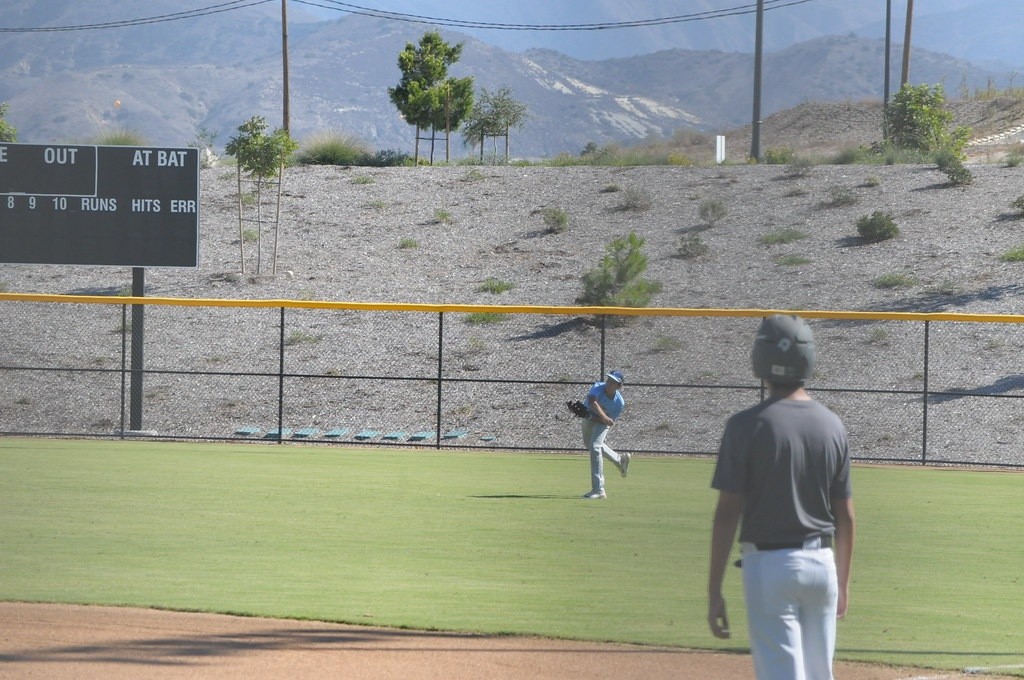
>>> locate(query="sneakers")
[620,452,630,478]
[584,492,606,499]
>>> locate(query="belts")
[756,537,832,551]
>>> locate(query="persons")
[708,314,852,680]
[568,371,631,498]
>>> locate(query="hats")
[606,370,624,385]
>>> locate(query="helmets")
[750,314,816,383]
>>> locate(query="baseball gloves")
[565,399,593,421]
